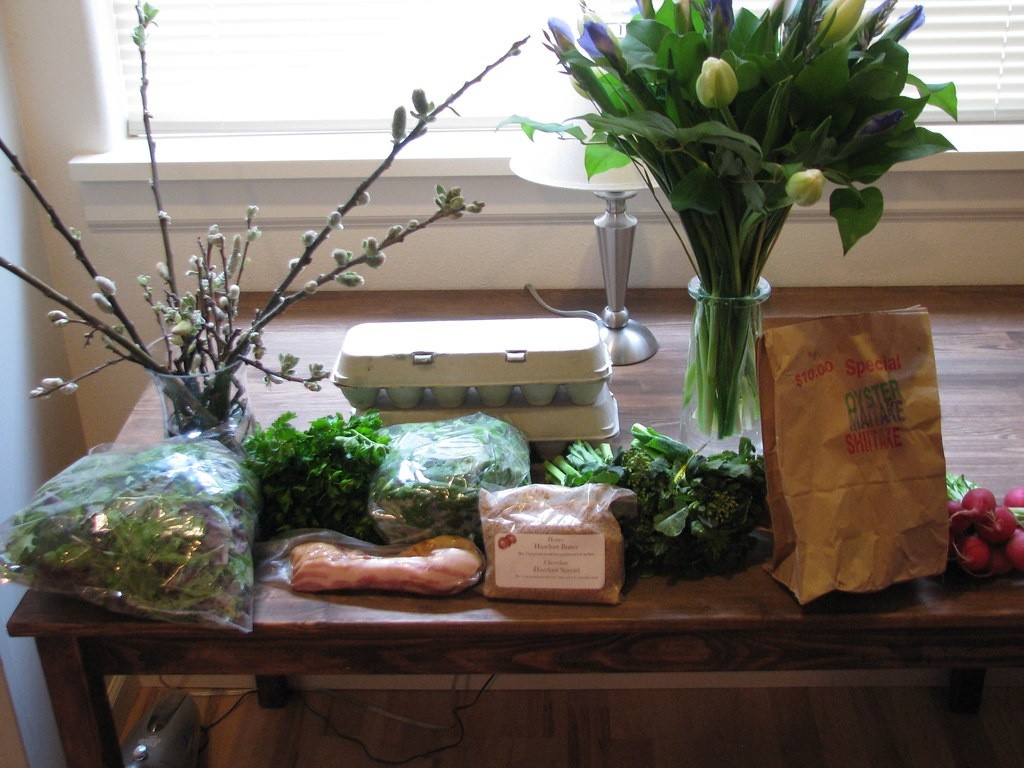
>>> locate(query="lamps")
[509,74,661,366]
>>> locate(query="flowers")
[539,1,957,290]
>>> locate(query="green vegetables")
[544,421,766,578]
[2,408,531,629]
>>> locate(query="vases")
[681,274,774,461]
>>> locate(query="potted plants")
[0,2,534,456]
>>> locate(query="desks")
[5,282,1023,767]
